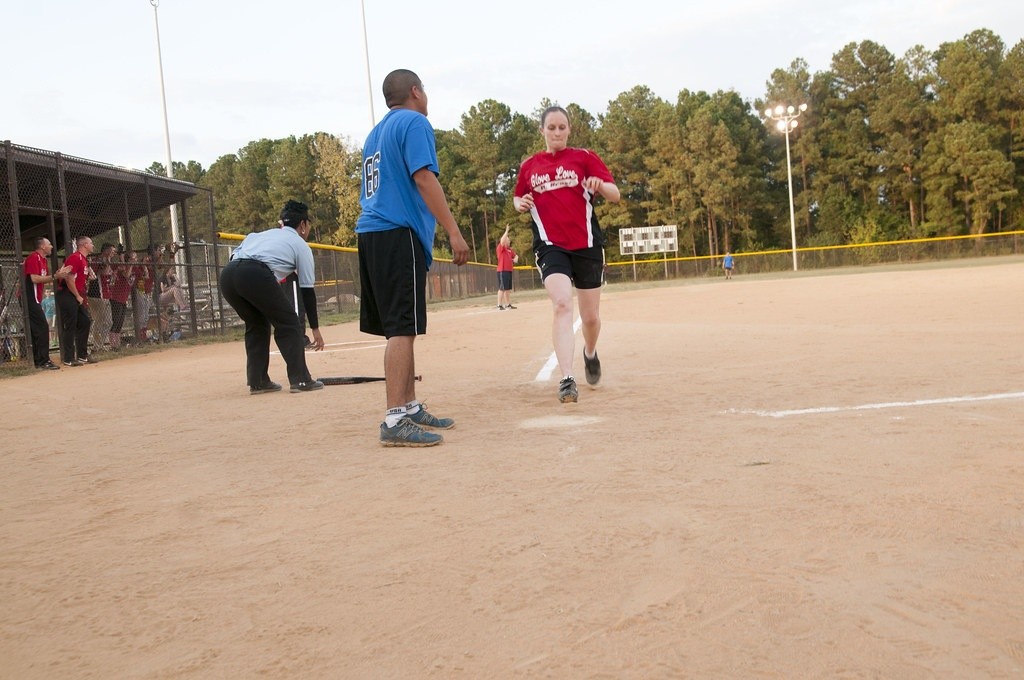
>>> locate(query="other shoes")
[180,307,191,312]
[54,342,58,346]
[163,338,171,343]
[90,349,108,354]
[50,340,55,347]
[11,356,16,361]
[304,343,316,351]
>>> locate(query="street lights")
[765,104,808,271]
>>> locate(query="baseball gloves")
[164,241,181,258]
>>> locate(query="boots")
[109,332,122,352]
[140,327,148,342]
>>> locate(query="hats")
[284,199,311,222]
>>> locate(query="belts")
[230,259,263,264]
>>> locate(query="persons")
[722,251,734,279]
[496,224,519,310]
[0,236,191,369]
[219,199,325,392]
[354,70,470,449]
[514,107,621,404]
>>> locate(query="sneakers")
[505,304,517,309]
[41,361,60,370]
[78,356,98,363]
[378,417,443,447]
[64,360,83,367]
[405,398,455,430]
[558,375,579,402]
[582,344,603,389]
[248,381,282,395]
[47,359,54,363]
[497,305,506,310]
[288,379,324,393]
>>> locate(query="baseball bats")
[316,373,423,386]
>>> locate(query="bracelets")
[52,273,55,281]
